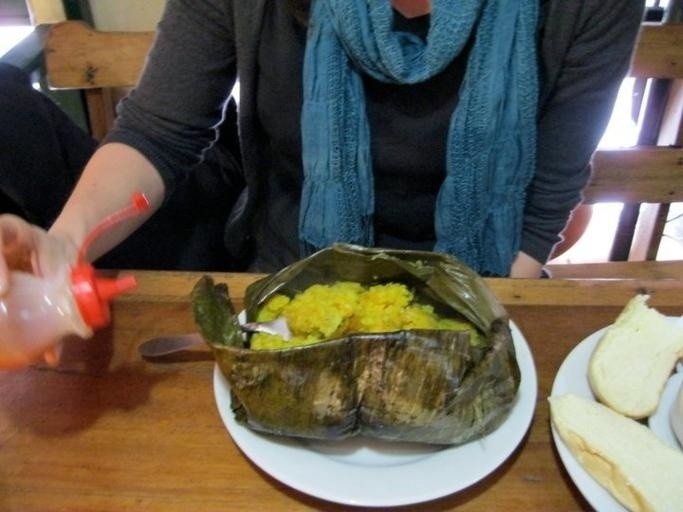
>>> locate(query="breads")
[546,293,683,512]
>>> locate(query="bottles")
[0,191,149,372]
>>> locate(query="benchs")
[42,19,683,280]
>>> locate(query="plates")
[549,317,683,512]
[212,304,539,507]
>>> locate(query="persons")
[0,1,646,371]
